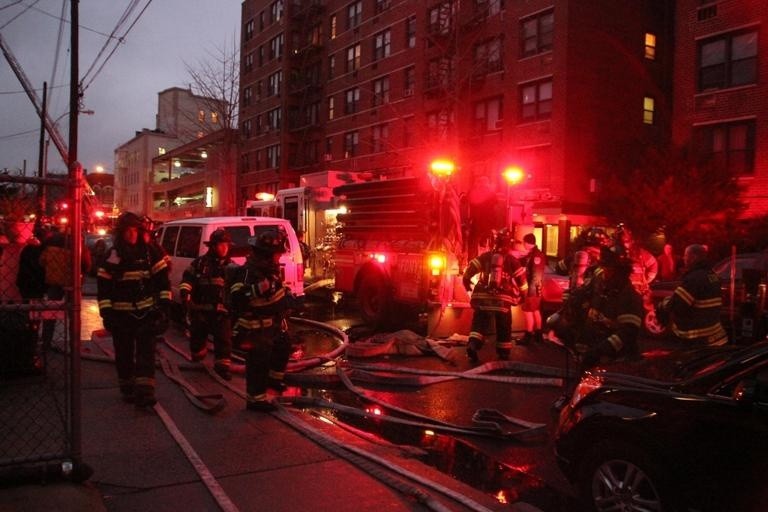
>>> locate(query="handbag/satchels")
[45,245,73,287]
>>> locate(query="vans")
[156,215,306,314]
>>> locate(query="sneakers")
[123,388,157,408]
[264,376,286,391]
[514,337,530,344]
[246,400,279,413]
[500,353,511,361]
[214,366,232,381]
[466,345,479,367]
[535,336,541,343]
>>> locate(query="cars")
[549,336,767,511]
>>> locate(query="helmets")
[573,227,616,257]
[495,227,517,246]
[115,212,155,237]
[256,231,290,260]
[210,230,232,244]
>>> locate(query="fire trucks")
[243,160,534,322]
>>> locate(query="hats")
[523,233,536,245]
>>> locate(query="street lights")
[38,110,98,216]
